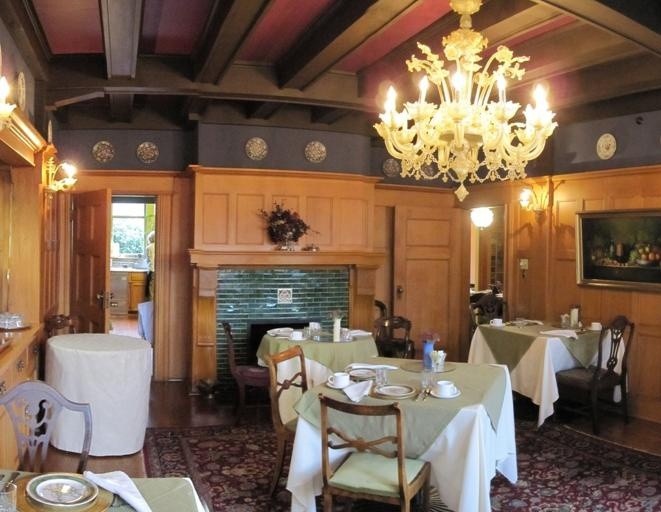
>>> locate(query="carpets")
[141,412,661,512]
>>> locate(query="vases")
[273,236,294,251]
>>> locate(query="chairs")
[43,313,77,338]
[0,380,92,473]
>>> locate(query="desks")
[43,333,154,459]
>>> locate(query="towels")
[82,470,154,512]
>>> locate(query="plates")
[349,368,377,379]
[374,384,416,398]
[26,471,99,506]
[327,380,355,389]
[587,326,602,331]
[489,323,506,327]
[429,388,462,399]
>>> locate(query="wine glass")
[420,368,431,398]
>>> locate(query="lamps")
[520,177,550,216]
[0,75,17,132]
[470,206,495,230]
[372,0,559,203]
[46,156,78,192]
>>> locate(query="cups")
[290,331,302,340]
[0,482,18,512]
[516,317,524,328]
[437,381,457,395]
[328,372,349,387]
[490,318,502,326]
[591,322,602,329]
[309,322,320,331]
[376,367,388,385]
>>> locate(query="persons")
[146,231,154,301]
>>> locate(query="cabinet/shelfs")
[0,320,45,471]
[127,271,148,312]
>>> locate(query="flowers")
[255,199,321,244]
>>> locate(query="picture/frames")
[574,207,661,293]
